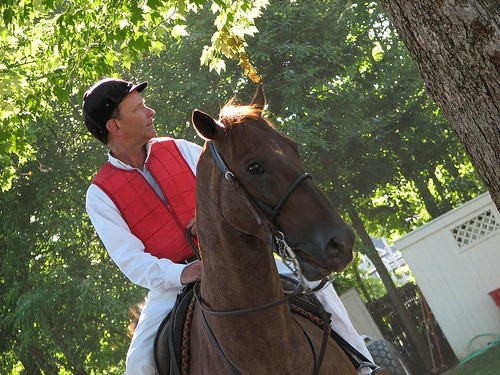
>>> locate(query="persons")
[81,78,380,375]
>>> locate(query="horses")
[156,84,360,375]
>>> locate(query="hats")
[81,78,149,144]
